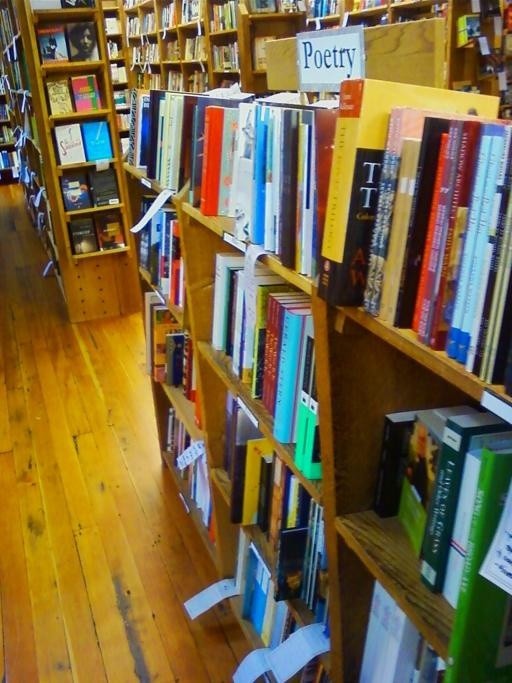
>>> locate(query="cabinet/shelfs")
[111,0,511,683]
[1,1,140,314]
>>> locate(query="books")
[360,404,511,682]
[122,1,512,397]
[2,1,127,255]
[137,292,328,683]
